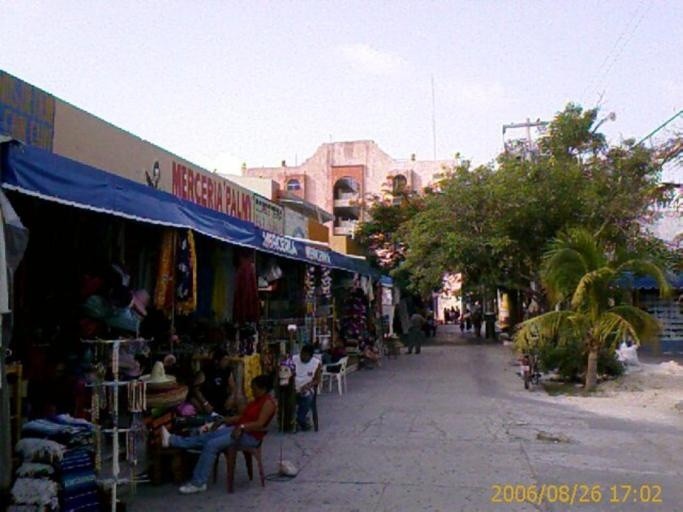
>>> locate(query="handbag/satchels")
[110,308,141,331]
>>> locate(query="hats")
[130,289,151,315]
[139,360,177,388]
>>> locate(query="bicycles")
[519,348,541,390]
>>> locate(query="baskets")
[128,386,189,407]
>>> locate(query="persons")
[473,308,483,337]
[277,344,321,433]
[425,311,437,337]
[367,300,379,337]
[162,350,276,493]
[443,305,460,324]
[460,310,472,333]
[405,309,421,354]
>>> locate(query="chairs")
[317,355,349,396]
[213,426,271,494]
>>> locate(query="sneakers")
[160,424,172,448]
[179,483,207,494]
